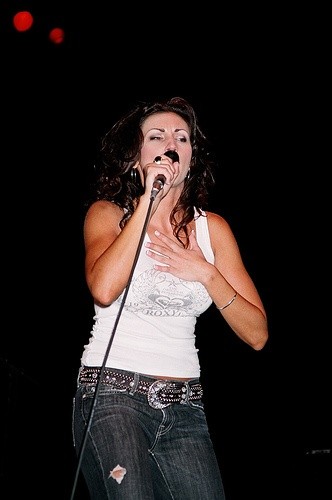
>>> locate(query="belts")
[76,367,205,410]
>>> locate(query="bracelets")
[215,291,237,310]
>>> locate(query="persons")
[71,98,270,500]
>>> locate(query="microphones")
[150,151,179,198]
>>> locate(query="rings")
[152,155,162,165]
